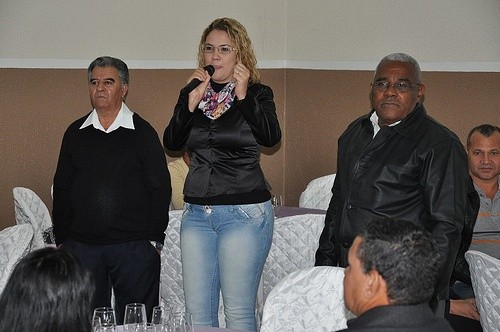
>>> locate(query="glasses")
[371,81,420,93]
[200,43,236,55]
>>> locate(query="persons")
[0,248,96,332]
[333,216,456,332]
[52,57,173,324]
[314,52,480,308]
[164,17,282,332]
[449,124,500,321]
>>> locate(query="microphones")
[182,64,215,94]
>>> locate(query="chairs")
[158,173,356,332]
[0,186,55,297]
[464,250,500,332]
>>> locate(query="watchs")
[150,241,163,250]
